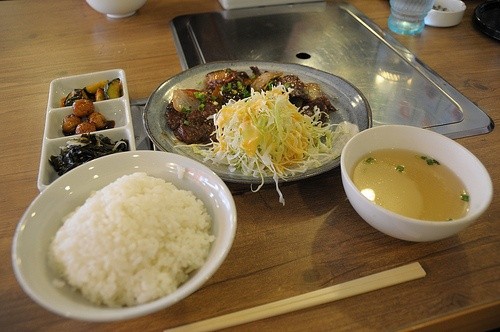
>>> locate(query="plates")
[142,60,373,183]
[37,68,136,192]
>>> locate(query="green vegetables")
[193,85,346,205]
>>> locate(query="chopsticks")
[163,262,426,332]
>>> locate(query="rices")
[46,172,214,307]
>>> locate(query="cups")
[387,0,435,35]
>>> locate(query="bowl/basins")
[10,150,237,321]
[85,0,148,18]
[341,124,493,241]
